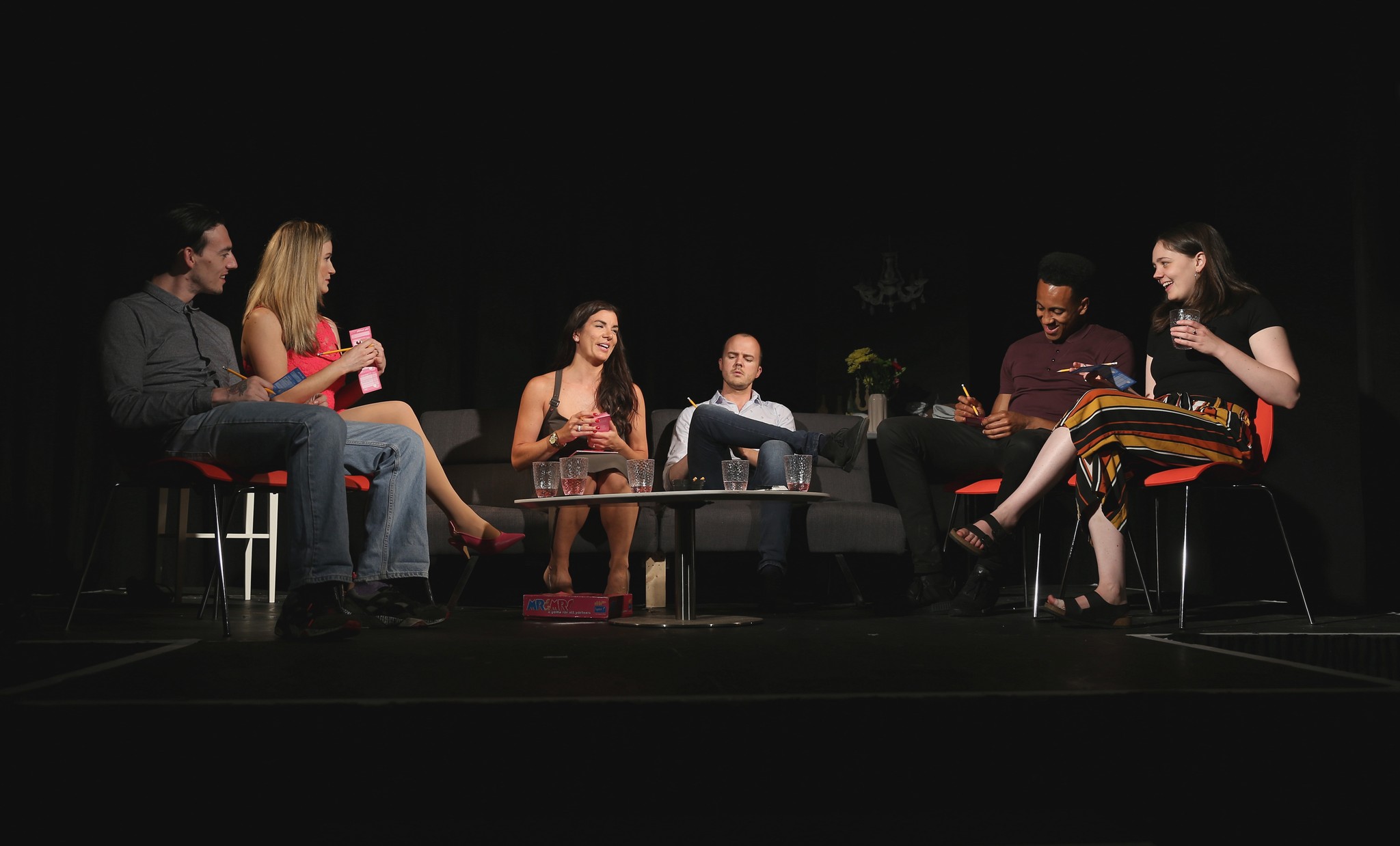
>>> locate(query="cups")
[1169,309,1201,349]
[722,460,749,490]
[559,457,588,495]
[533,462,560,498]
[627,459,655,493]
[783,455,813,492]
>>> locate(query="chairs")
[64,458,375,635]
[1067,398,1314,630]
[941,478,1030,607]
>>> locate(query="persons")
[876,248,1133,621]
[662,333,870,614]
[509,299,650,595]
[97,204,445,642]
[239,220,527,561]
[949,217,1301,630]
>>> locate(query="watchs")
[549,430,567,449]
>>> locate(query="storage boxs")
[522,591,633,619]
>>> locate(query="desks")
[514,490,829,629]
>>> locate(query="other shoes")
[871,572,954,618]
[948,565,1009,617]
[543,565,551,593]
[604,569,630,595]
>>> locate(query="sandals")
[1041,590,1133,628]
[948,514,1009,558]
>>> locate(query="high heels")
[448,519,526,559]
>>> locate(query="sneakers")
[274,591,361,639]
[342,582,450,627]
[818,417,869,474]
[757,570,798,617]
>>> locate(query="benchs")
[420,409,905,611]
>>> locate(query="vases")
[867,394,887,433]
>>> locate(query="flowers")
[844,347,906,393]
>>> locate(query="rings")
[1192,329,1197,335]
[593,443,596,448]
[578,425,581,432]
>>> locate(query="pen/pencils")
[580,417,584,421]
[1056,362,1117,372]
[317,344,378,356]
[222,366,276,395]
[687,397,697,408]
[961,384,979,416]
